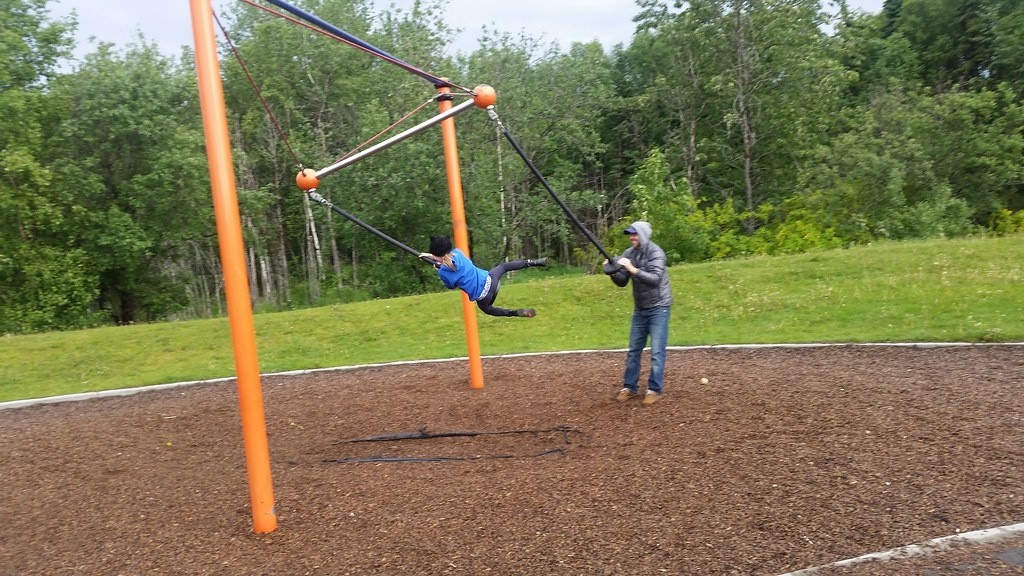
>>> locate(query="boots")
[508,308,537,317]
[527,257,548,267]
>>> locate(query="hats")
[429,235,452,256]
[623,227,637,235]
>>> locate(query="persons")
[417,235,548,318]
[601,221,672,405]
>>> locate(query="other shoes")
[642,393,664,405]
[616,389,640,402]
